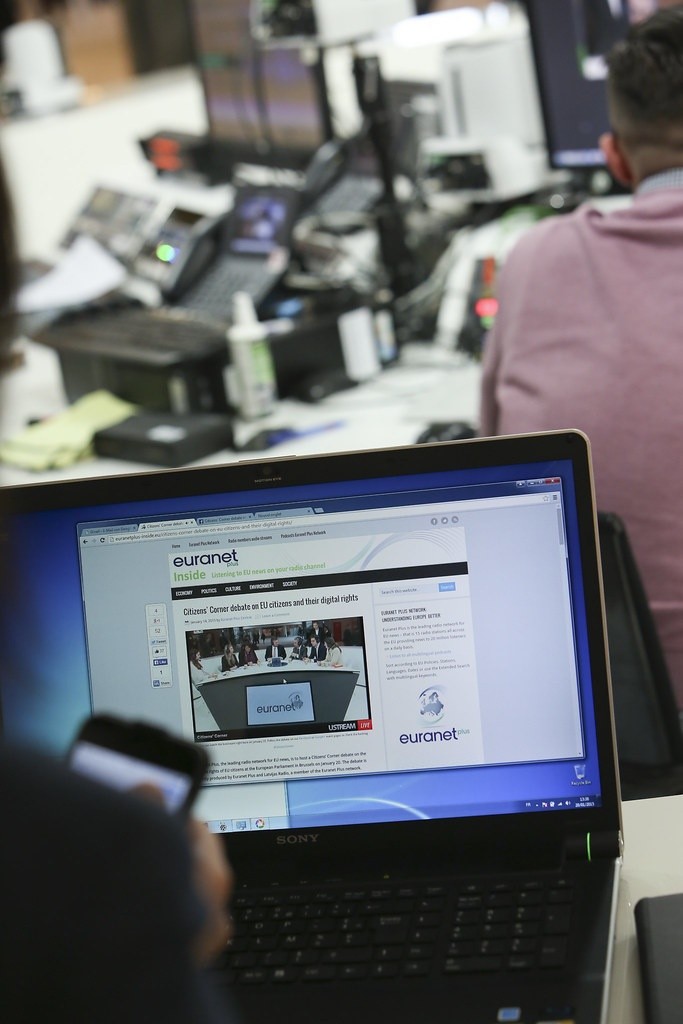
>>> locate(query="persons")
[238,643,258,667]
[480,0,683,728]
[289,622,341,665]
[265,639,287,661]
[189,649,214,691]
[221,645,238,673]
[1,725,237,1022]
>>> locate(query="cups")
[3,20,67,113]
[213,656,335,680]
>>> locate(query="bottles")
[225,290,279,421]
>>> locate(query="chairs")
[594,510,683,802]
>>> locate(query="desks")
[0,59,212,265]
[0,343,484,489]
[196,659,360,730]
